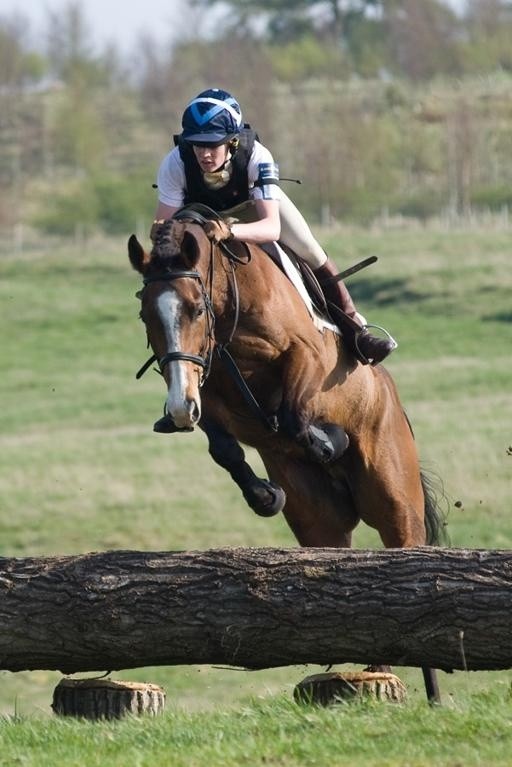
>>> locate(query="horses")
[127,216,452,710]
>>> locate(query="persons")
[150,88,398,433]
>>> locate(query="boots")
[311,250,394,365]
[152,413,194,434]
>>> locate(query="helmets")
[182,89,242,142]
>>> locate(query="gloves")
[150,218,167,241]
[205,218,234,241]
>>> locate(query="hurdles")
[0,546,510,718]
